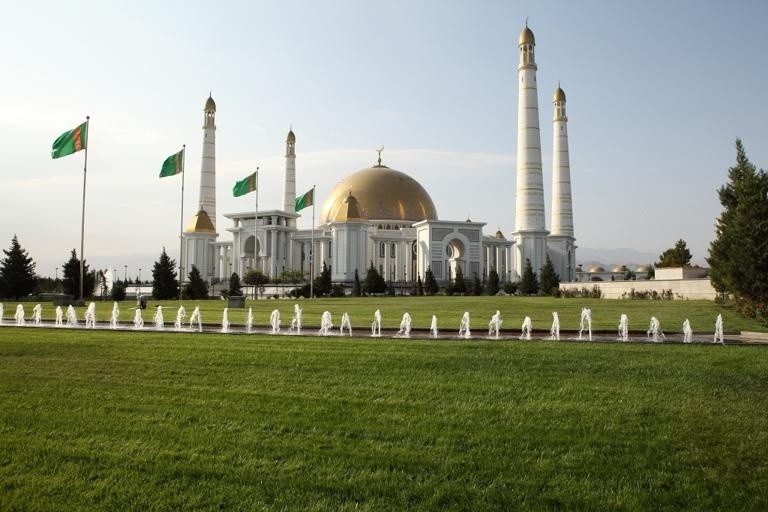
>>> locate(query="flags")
[157,148,184,179]
[294,188,313,213]
[50,120,89,160]
[232,171,257,198]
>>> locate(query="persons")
[140,293,147,309]
[135,293,142,309]
